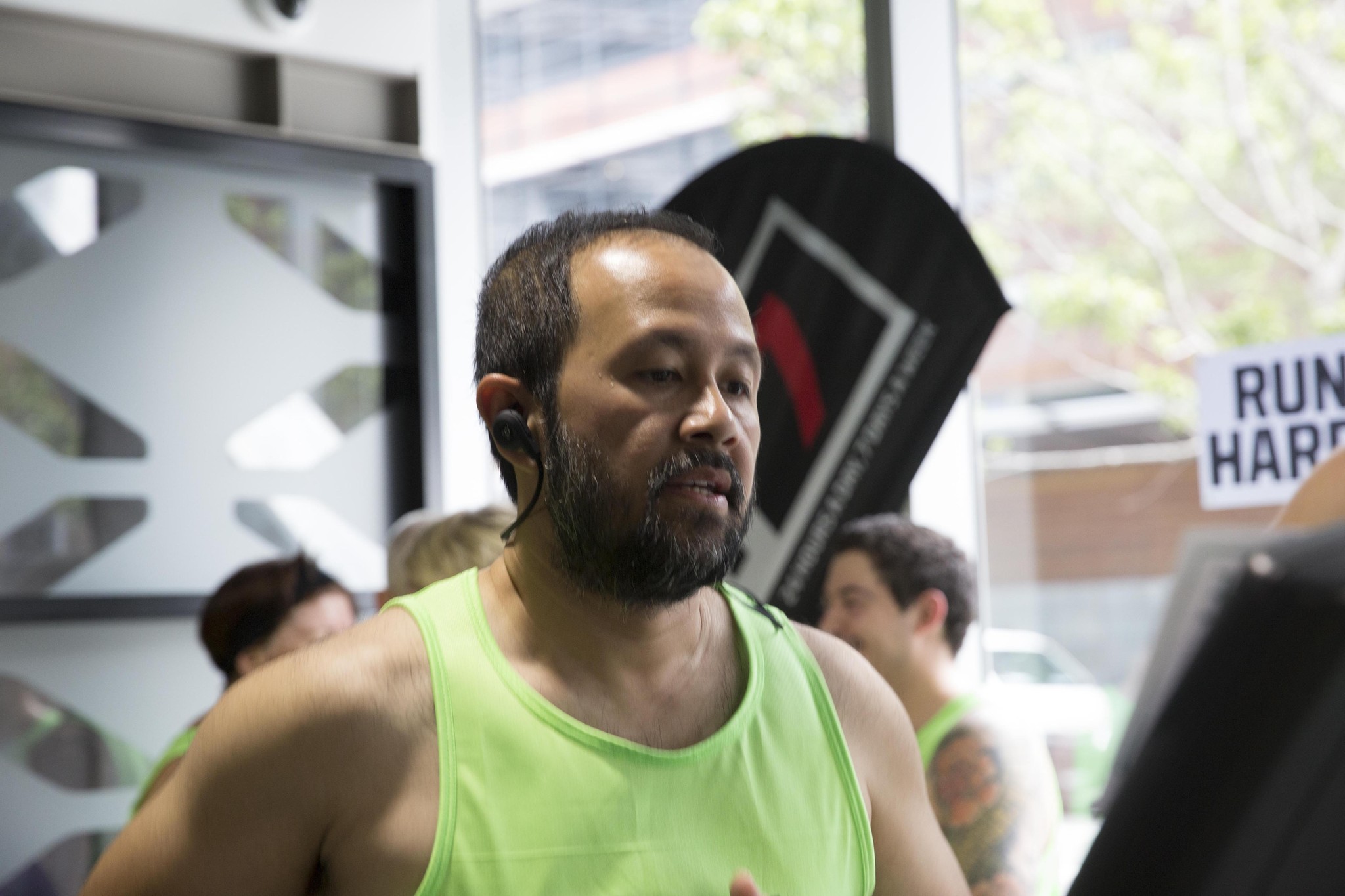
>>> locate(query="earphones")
[494,406,529,451]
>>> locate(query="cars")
[976,625,1136,816]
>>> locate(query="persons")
[76,205,974,896]
[132,497,1066,896]
